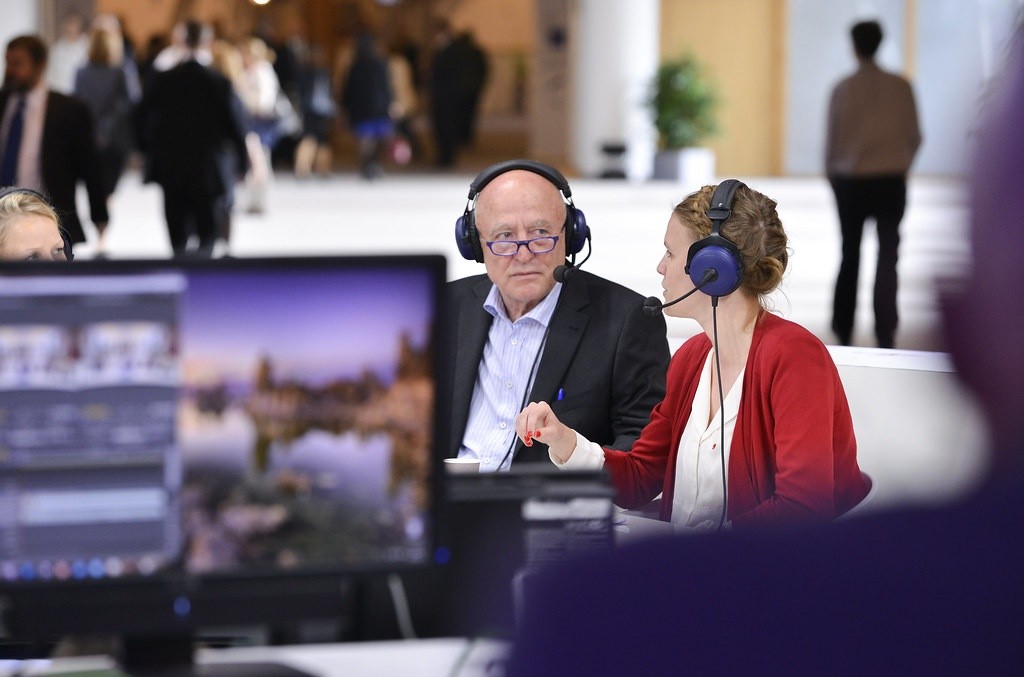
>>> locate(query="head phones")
[684,180,748,296]
[456,159,586,263]
[0,186,74,262]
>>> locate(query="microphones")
[643,268,718,317]
[553,226,591,283]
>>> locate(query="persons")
[39,0,495,216]
[0,183,69,269]
[523,25,571,170]
[821,19,924,352]
[428,158,669,467]
[124,16,255,263]
[0,35,110,264]
[513,180,875,535]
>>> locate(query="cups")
[445,456,481,477]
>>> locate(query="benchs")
[820,314,997,520]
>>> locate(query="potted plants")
[648,60,718,184]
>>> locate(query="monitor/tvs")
[0,257,446,639]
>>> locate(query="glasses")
[477,220,568,257]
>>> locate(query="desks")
[434,468,618,502]
[8,635,517,676]
